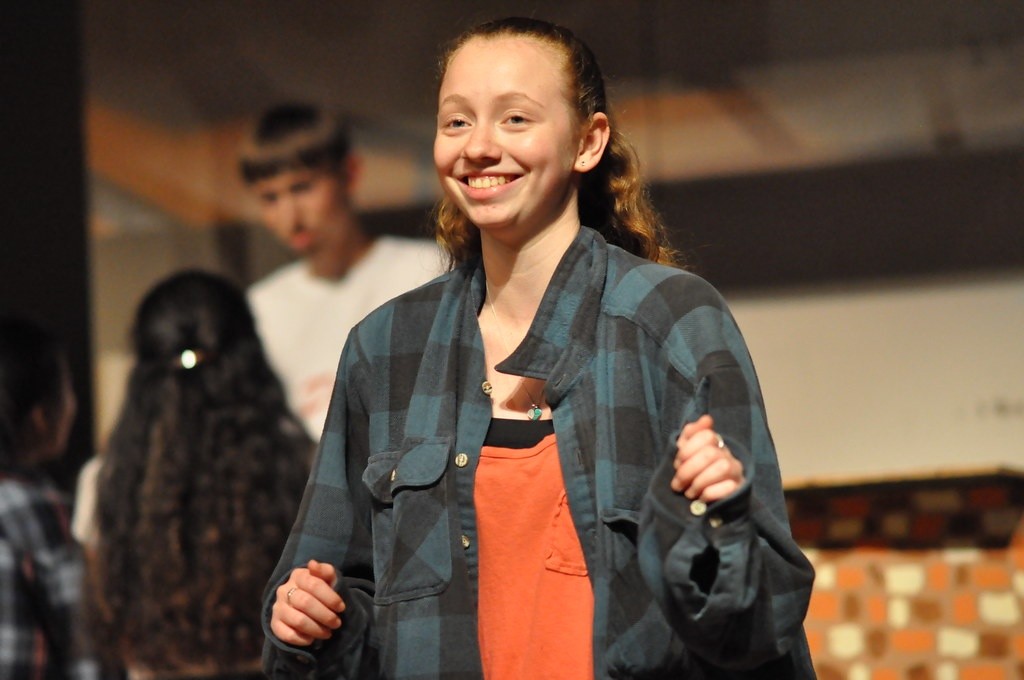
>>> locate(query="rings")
[716,430,726,446]
[285,586,297,601]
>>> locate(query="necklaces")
[484,273,548,420]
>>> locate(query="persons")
[0,263,319,680]
[234,93,457,446]
[254,14,819,679]
